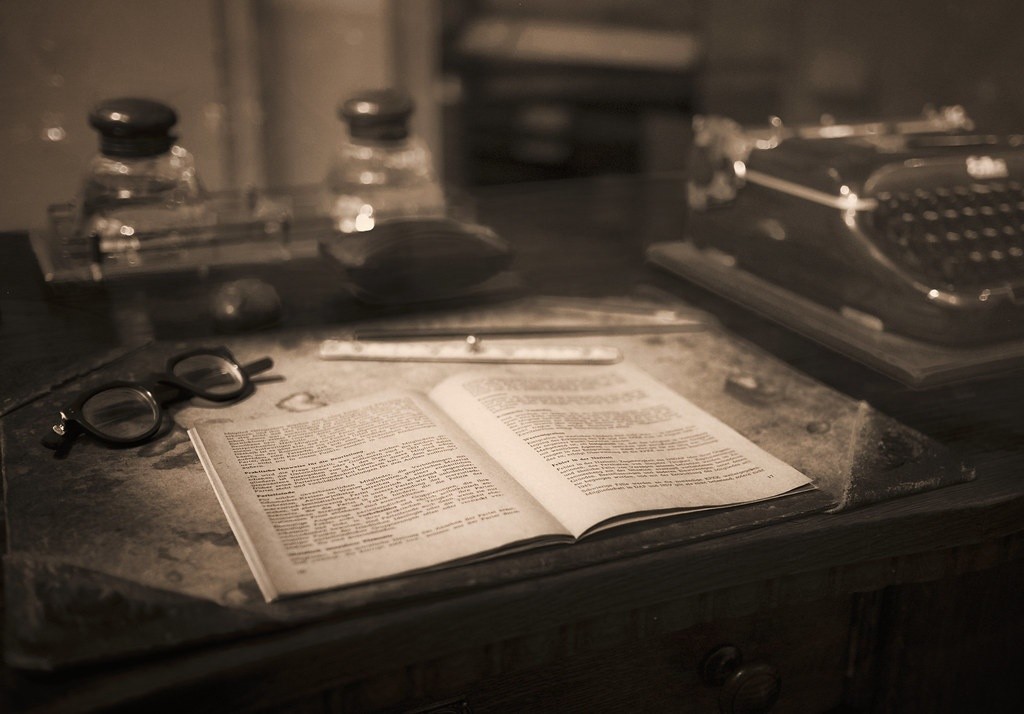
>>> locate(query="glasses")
[53,346,275,446]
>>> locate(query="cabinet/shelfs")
[0,168,1024,714]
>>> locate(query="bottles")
[323,89,448,235]
[69,97,217,256]
